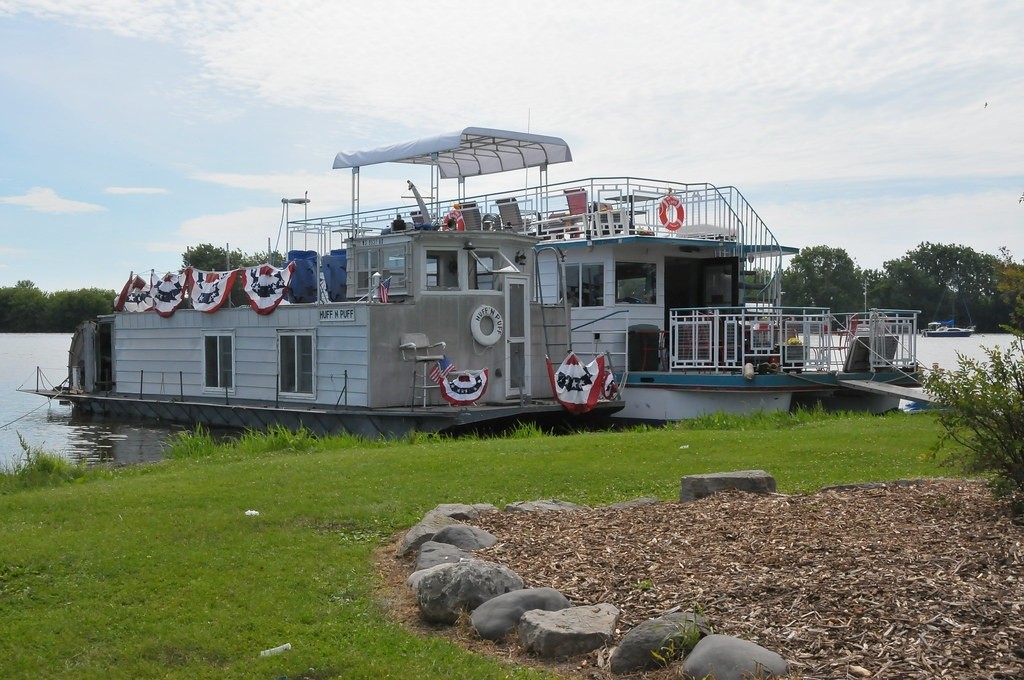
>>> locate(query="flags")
[430,355,456,384]
[379,278,391,303]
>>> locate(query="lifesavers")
[471,306,503,347]
[442,211,464,232]
[658,196,684,231]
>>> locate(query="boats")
[822,278,880,336]
[15,179,626,447]
[289,125,997,428]
[919,264,976,338]
